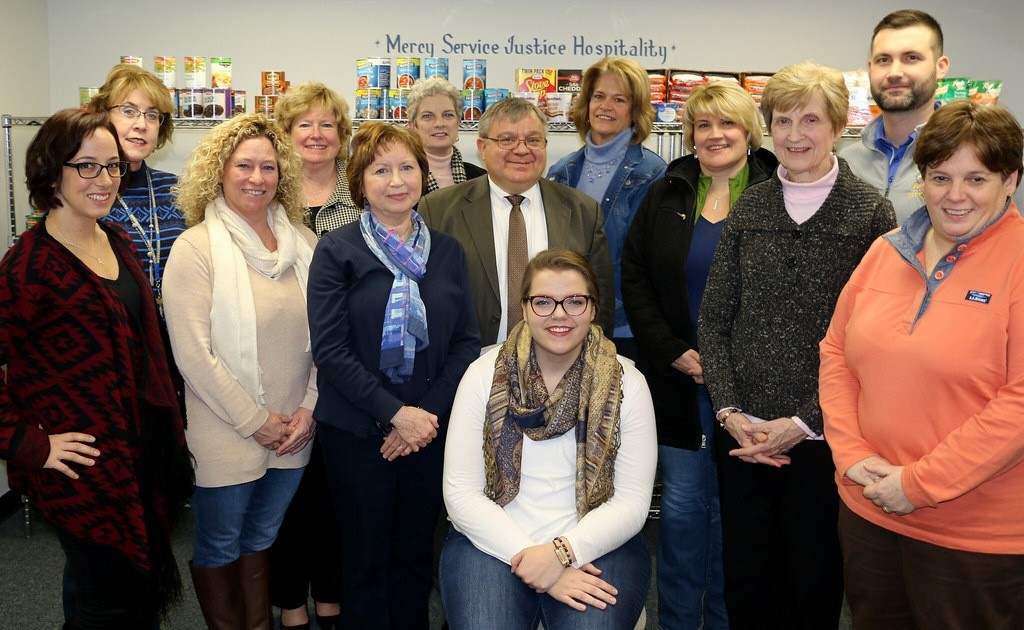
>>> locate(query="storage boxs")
[646,67,775,122]
[514,68,582,122]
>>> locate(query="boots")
[233,550,275,630]
[187,551,244,630]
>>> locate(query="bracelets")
[720,409,738,429]
[553,537,572,568]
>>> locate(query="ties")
[504,193,526,338]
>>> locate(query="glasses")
[107,103,165,127]
[481,133,548,149]
[59,159,130,179]
[518,292,595,318]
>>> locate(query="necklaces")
[310,175,335,200]
[708,191,725,210]
[585,151,625,182]
[115,166,163,306]
[46,216,103,265]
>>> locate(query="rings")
[884,506,888,513]
[400,446,404,451]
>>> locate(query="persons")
[162,113,318,630]
[622,80,778,630]
[307,121,481,630]
[419,98,615,341]
[0,109,194,630]
[438,247,658,630]
[838,10,950,226]
[273,80,363,630]
[87,63,188,315]
[407,77,488,197]
[819,98,1024,630]
[696,62,897,630]
[547,57,668,337]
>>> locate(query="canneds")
[355,57,510,122]
[78,55,246,120]
[254,70,291,121]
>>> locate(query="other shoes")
[647,482,662,520]
[279,607,311,630]
[314,599,343,630]
